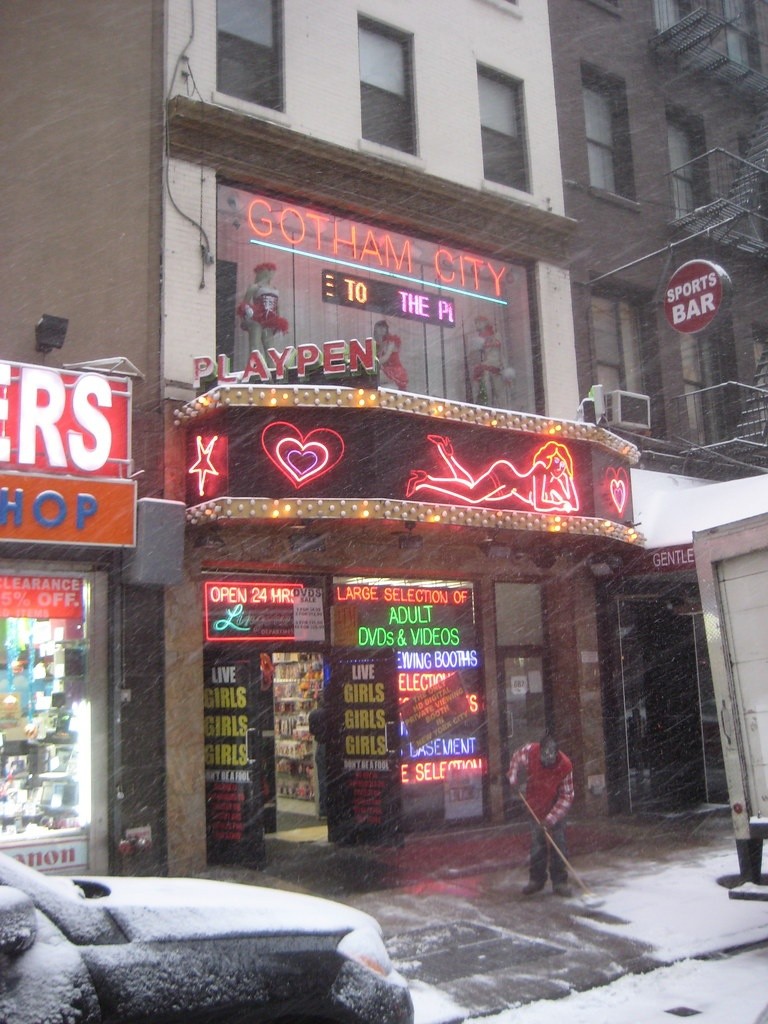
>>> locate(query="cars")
[0,852,417,1024]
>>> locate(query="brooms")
[517,792,610,909]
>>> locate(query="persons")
[471,315,512,410]
[505,735,576,897]
[372,320,409,390]
[237,262,289,369]
[308,692,328,819]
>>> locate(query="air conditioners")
[603,388,653,431]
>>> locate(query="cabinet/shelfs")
[27,739,79,817]
[276,660,320,819]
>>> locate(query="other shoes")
[523,878,545,895]
[552,882,572,897]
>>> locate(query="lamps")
[397,522,425,553]
[585,547,615,578]
[477,529,510,558]
[35,312,70,351]
[192,525,225,551]
[288,518,326,553]
[90,556,112,570]
[573,382,608,426]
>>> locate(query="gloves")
[501,775,519,794]
[536,821,554,845]
